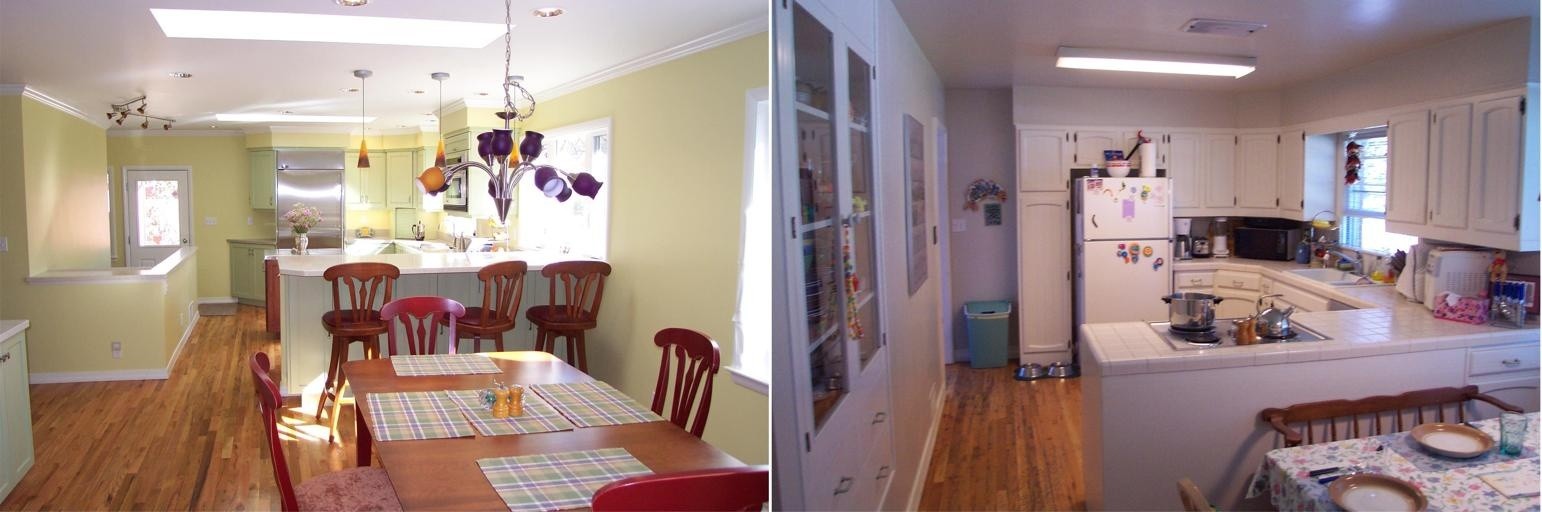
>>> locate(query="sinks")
[1282,267,1398,289]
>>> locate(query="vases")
[291,233,309,255]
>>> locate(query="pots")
[1161,292,1223,330]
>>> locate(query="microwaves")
[1234,219,1302,260]
[442,150,469,213]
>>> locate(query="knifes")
[1389,248,1407,273]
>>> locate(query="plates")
[1410,422,1495,460]
[1327,472,1429,512]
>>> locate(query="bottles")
[509,385,525,416]
[1492,281,1524,327]
[493,382,509,418]
[1091,162,1099,177]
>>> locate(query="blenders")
[1211,217,1231,258]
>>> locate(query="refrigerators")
[1073,176,1173,366]
[275,150,345,248]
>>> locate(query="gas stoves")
[1146,317,1334,351]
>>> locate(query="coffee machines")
[1175,217,1195,260]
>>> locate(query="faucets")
[1322,249,1364,276]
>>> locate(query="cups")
[412,220,425,241]
[1499,413,1528,456]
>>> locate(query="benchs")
[1261,384,1524,449]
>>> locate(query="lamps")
[432,72,450,168]
[354,70,373,167]
[418,1,603,225]
[1054,45,1256,79]
[106,96,176,130]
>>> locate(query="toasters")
[1192,235,1212,259]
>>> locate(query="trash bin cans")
[964,300,1011,368]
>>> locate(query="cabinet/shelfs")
[344,127,514,217]
[248,151,277,209]
[1465,342,1540,421]
[0,320,36,505]
[776,0,898,511]
[229,243,275,308]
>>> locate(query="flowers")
[283,202,325,233]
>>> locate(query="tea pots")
[1253,293,1297,338]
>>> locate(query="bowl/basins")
[1017,363,1043,380]
[1105,159,1132,177]
[1047,362,1074,378]
